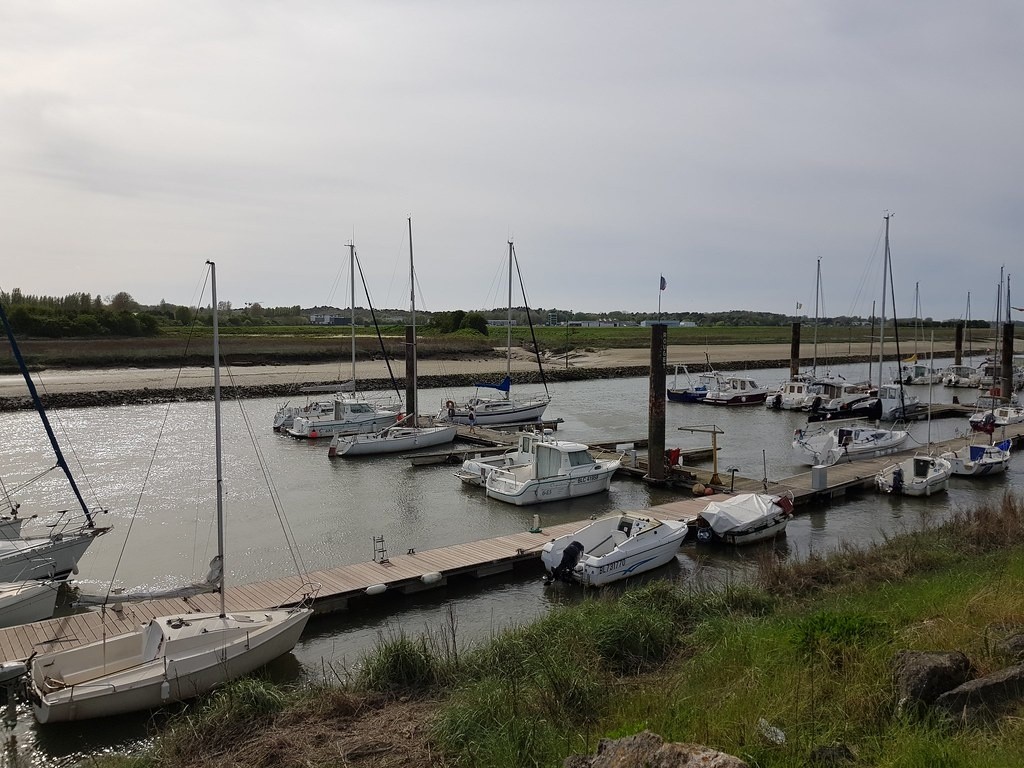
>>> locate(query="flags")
[660,277,667,290]
[796,302,802,309]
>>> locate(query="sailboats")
[272,243,557,459]
[27,259,318,727]
[667,212,1024,498]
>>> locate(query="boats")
[0,303,114,628]
[695,489,795,547]
[452,429,622,507]
[792,418,914,466]
[540,513,689,587]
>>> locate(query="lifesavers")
[446,401,454,408]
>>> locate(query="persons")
[468,408,477,434]
